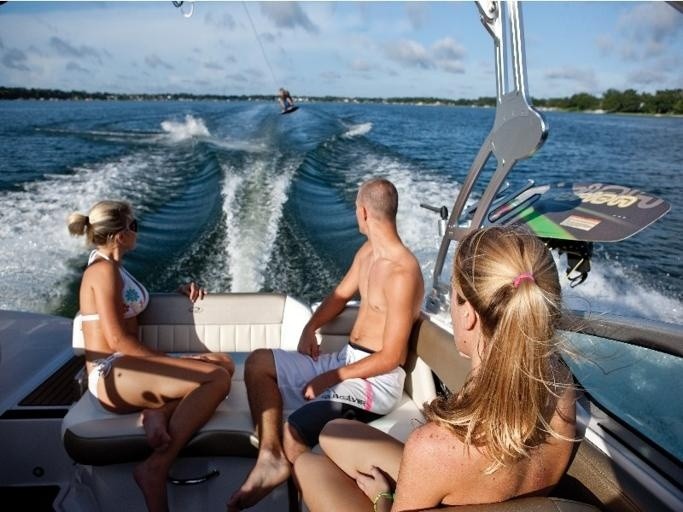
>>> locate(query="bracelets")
[372,490,393,512]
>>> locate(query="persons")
[68,199,235,511]
[288,222,580,512]
[225,176,425,512]
[277,87,293,113]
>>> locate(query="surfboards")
[280,106,299,115]
[438,182,671,243]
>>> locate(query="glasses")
[129,219,138,232]
